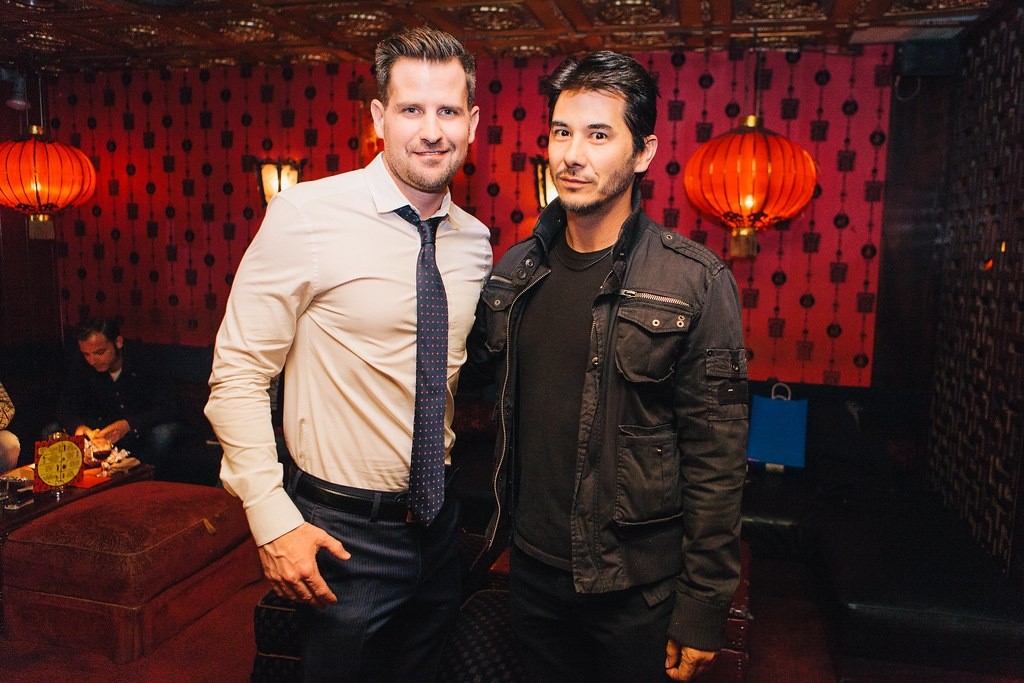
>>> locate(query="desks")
[0,457,154,544]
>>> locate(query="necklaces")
[552,233,614,271]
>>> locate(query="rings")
[113,431,117,434]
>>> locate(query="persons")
[0,382,20,476]
[37,317,155,465]
[441,51,751,683]
[205,26,493,683]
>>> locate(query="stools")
[0,480,265,665]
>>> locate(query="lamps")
[254,156,307,209]
[529,153,559,213]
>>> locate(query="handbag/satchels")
[746,383,808,469]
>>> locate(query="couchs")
[0,341,288,488]
[739,378,1024,683]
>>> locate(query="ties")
[395,206,449,527]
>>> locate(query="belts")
[287,464,422,523]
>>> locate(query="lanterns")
[0,125,97,240]
[683,116,816,260]
